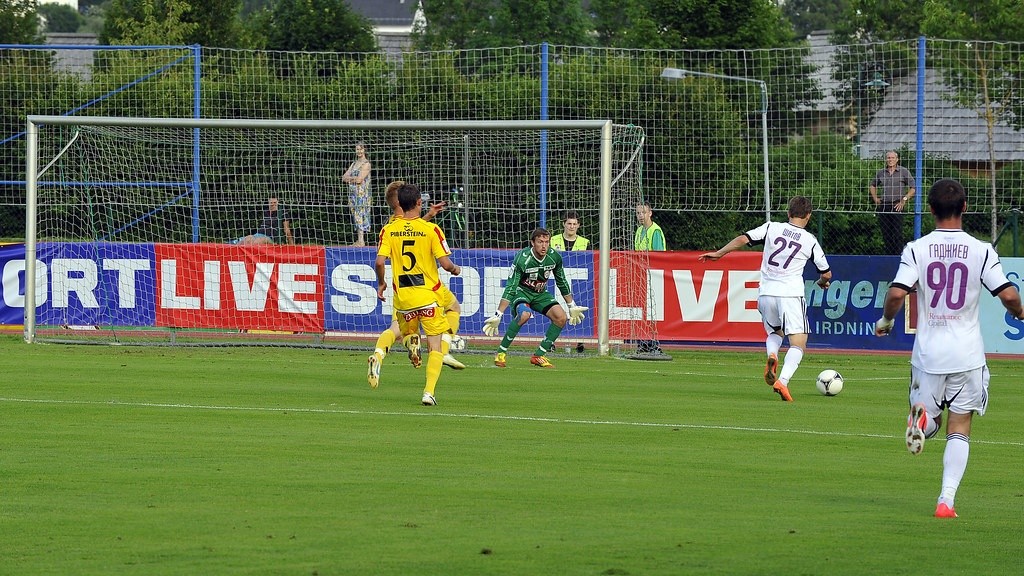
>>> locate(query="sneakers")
[934,503,958,518]
[764,353,778,385]
[530,353,552,368]
[774,379,793,402]
[420,392,438,406]
[367,354,381,388]
[442,353,465,370]
[408,334,423,369]
[905,403,927,455]
[494,352,507,367]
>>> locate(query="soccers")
[815,369,845,396]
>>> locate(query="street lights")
[659,66,769,224]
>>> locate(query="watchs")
[903,197,907,201]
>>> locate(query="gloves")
[483,310,503,337]
[567,301,589,326]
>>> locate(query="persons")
[482,227,589,369]
[548,212,592,353]
[870,150,916,256]
[698,196,831,403]
[225,195,294,244]
[876,180,1023,519]
[366,181,465,405]
[342,141,372,247]
[634,200,666,353]
